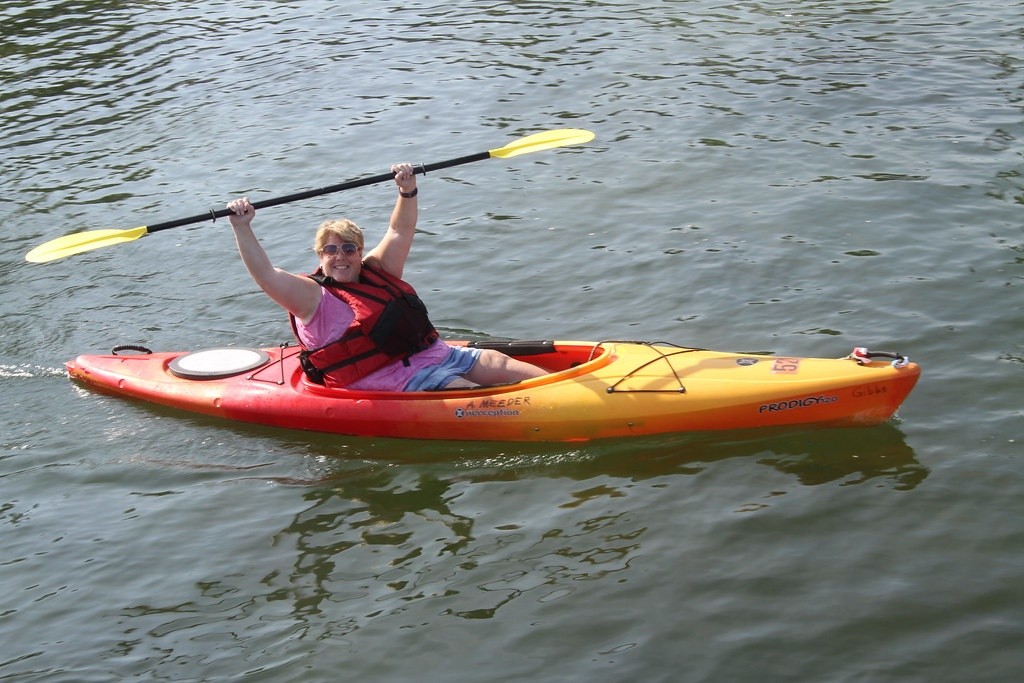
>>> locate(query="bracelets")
[399,186,417,198]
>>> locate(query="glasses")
[320,243,360,256]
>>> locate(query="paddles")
[20,126,599,266]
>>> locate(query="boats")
[62,343,921,441]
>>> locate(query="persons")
[227,163,552,391]
